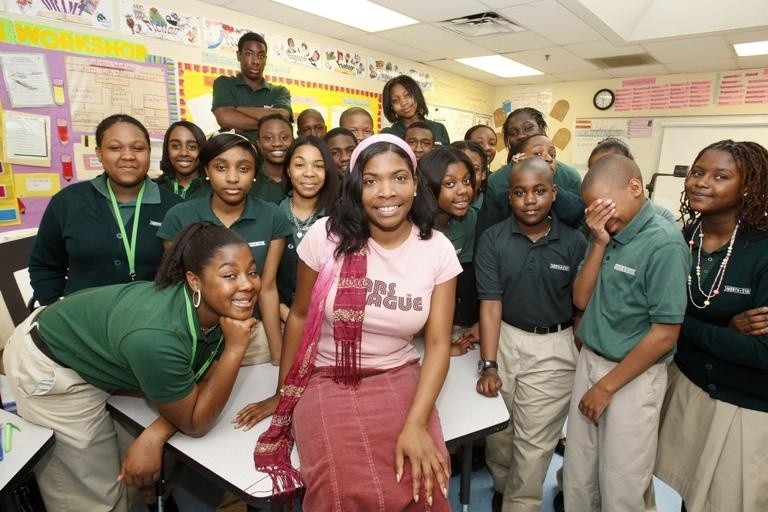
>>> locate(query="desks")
[102,339,512,510]
[0,408,54,511]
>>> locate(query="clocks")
[592,87,615,110]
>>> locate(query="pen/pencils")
[0,423,21,461]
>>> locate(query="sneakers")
[550,435,570,512]
[448,446,488,477]
[491,487,511,512]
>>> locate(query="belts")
[29,304,73,369]
[501,315,573,335]
